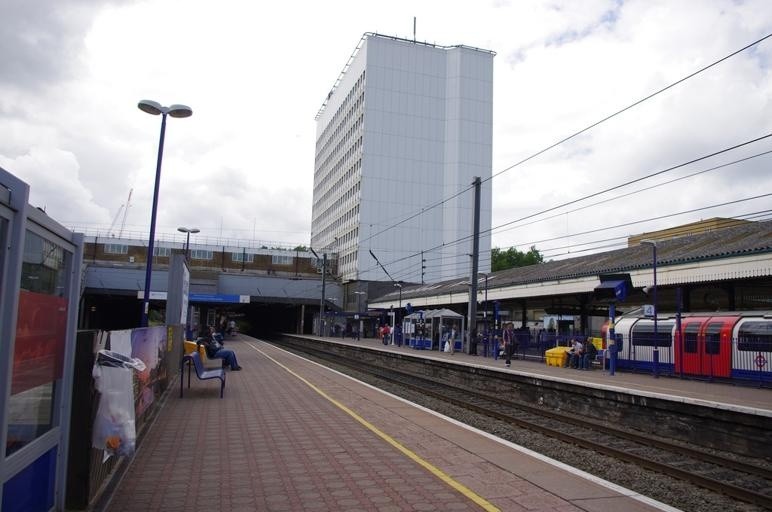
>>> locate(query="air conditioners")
[316,267,333,274]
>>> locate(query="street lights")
[394,284,401,347]
[177,226,201,260]
[478,272,488,357]
[354,292,365,341]
[329,298,337,336]
[640,239,659,378]
[138,100,192,329]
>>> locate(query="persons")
[198,318,242,371]
[330,320,599,372]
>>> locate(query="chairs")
[180,351,226,399]
[593,348,608,370]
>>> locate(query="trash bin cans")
[184,341,206,363]
[545,347,573,368]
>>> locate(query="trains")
[600,311,772,386]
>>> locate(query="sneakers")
[230,365,242,371]
[563,363,590,371]
[504,363,511,369]
